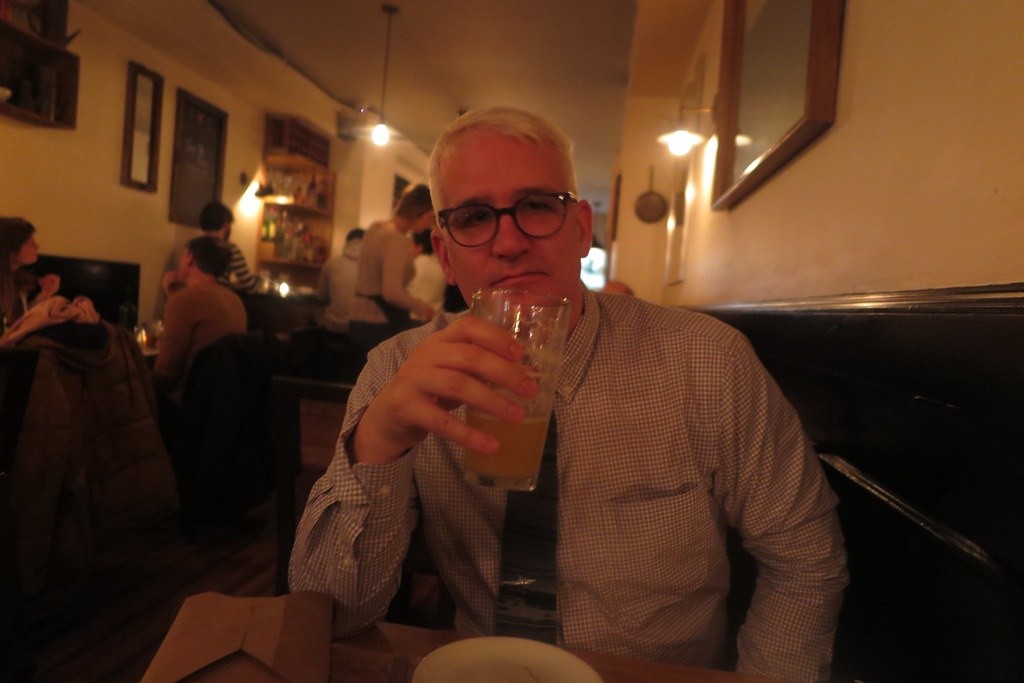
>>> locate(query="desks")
[328,618,774,683]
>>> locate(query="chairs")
[1,331,455,632]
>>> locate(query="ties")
[493,409,560,648]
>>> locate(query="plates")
[412,636,603,683]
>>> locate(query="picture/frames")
[711,0,845,214]
[119,60,164,193]
[169,87,230,229]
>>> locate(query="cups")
[144,321,161,349]
[458,287,572,493]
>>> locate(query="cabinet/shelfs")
[255,111,339,280]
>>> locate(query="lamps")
[372,6,400,147]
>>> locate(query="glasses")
[438,189,576,247]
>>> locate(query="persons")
[293,228,366,397]
[0,217,60,352]
[288,104,847,683]
[348,185,445,383]
[152,236,248,430]
[156,202,261,322]
